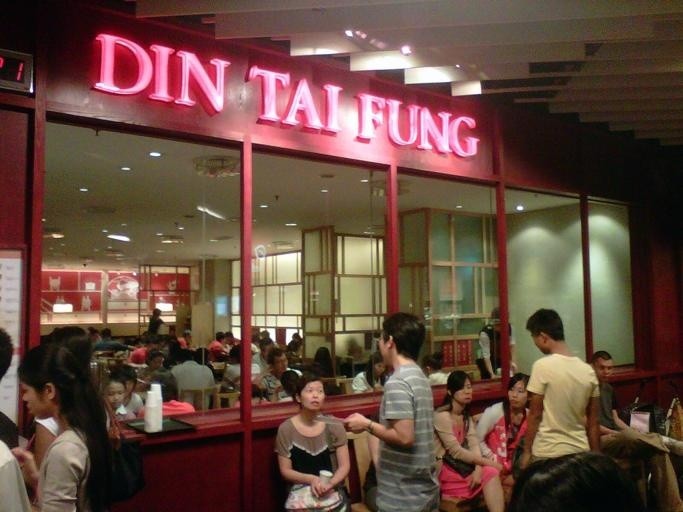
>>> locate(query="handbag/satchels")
[443,438,475,478]
[111,438,144,502]
[284,483,348,512]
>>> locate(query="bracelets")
[367,419,374,435]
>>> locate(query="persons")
[274,373,352,512]
[516,306,601,474]
[88,306,449,422]
[476,308,518,379]
[432,369,505,512]
[588,349,682,512]
[341,311,442,512]
[474,373,531,504]
[0,323,115,512]
[509,451,648,512]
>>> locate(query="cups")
[319,470,333,487]
[144,383,163,432]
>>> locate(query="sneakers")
[667,438,683,456]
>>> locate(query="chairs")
[92,357,358,411]
